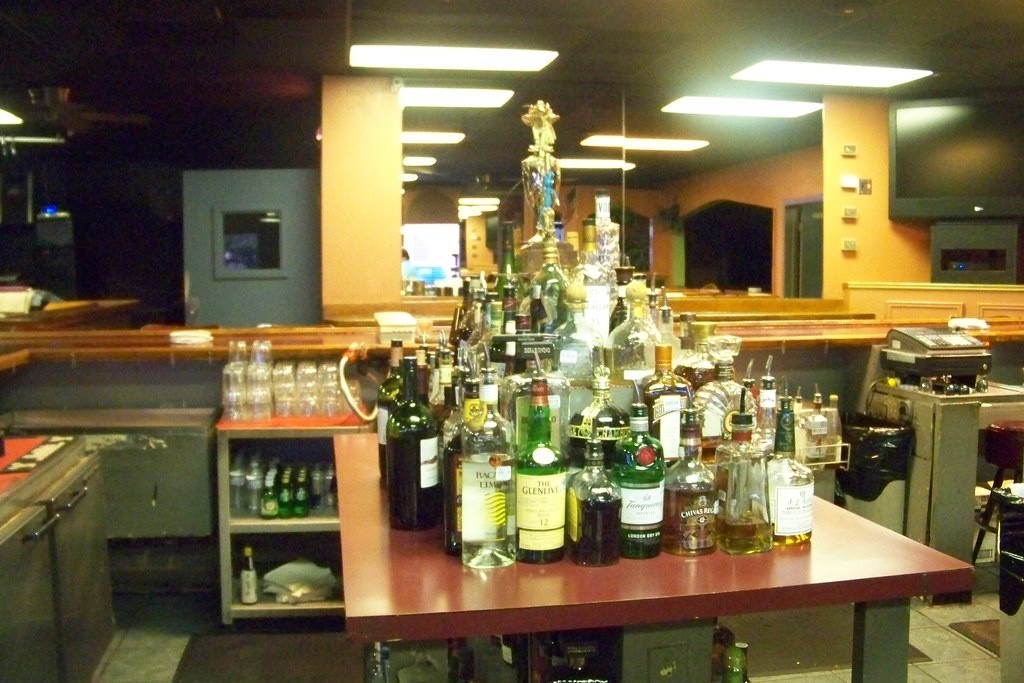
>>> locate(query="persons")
[402,249,412,278]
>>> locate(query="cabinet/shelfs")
[217,416,377,625]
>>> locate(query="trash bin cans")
[834,409,911,536]
[991,482,1024,683]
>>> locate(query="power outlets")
[647,644,689,683]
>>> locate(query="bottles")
[721,643,751,683]
[230,451,337,518]
[241,546,257,604]
[377,189,843,567]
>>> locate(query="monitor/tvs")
[886,94,1024,220]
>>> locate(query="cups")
[223,339,362,421]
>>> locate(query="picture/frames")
[213,203,289,280]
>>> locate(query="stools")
[972,419,1024,564]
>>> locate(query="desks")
[332,431,977,683]
